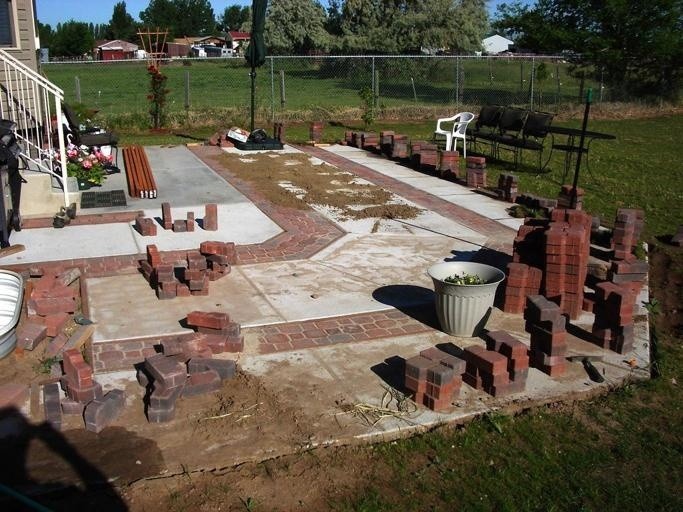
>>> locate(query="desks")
[535,127,616,187]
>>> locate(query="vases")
[77,178,91,190]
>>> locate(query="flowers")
[39,144,113,185]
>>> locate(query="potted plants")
[427,261,506,338]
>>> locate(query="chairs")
[466,105,504,161]
[515,110,555,173]
[433,111,475,159]
[0,270,24,359]
[62,103,118,168]
[488,106,530,169]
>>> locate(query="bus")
[203,46,233,57]
